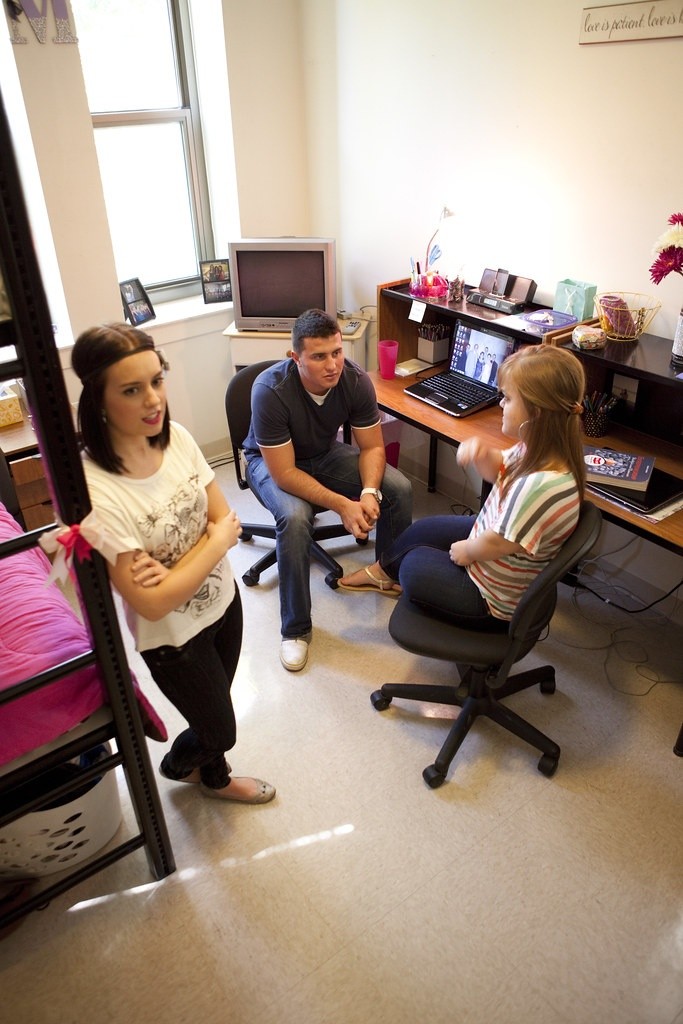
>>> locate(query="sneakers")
[279,640,309,671]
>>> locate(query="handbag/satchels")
[553,279,597,322]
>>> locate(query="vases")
[669,308,683,371]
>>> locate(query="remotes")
[341,321,361,335]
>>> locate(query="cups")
[378,339,399,379]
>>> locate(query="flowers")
[649,212,683,285]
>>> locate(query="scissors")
[429,245,441,268]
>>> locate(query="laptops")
[404,318,520,419]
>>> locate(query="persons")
[70,324,277,805]
[465,344,498,385]
[241,308,414,671]
[204,264,225,282]
[132,306,151,323]
[328,337,587,632]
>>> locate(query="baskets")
[0,741,123,882]
[593,292,662,342]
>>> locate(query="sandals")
[337,566,403,600]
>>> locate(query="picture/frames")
[200,259,233,304]
[119,277,156,328]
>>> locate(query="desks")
[222,313,373,448]
[354,362,683,757]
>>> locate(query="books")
[582,445,657,493]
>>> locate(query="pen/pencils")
[583,390,617,413]
[418,323,451,341]
[417,262,422,285]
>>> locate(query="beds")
[0,455,175,938]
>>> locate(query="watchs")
[360,487,383,504]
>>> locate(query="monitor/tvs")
[228,236,337,332]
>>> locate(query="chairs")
[371,500,603,788]
[224,360,368,589]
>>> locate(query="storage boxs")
[417,336,449,364]
[552,278,597,322]
[0,737,123,880]
[0,387,24,427]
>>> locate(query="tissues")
[0,379,24,428]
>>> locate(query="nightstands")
[0,383,87,565]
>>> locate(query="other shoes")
[199,777,276,804]
[159,762,201,783]
